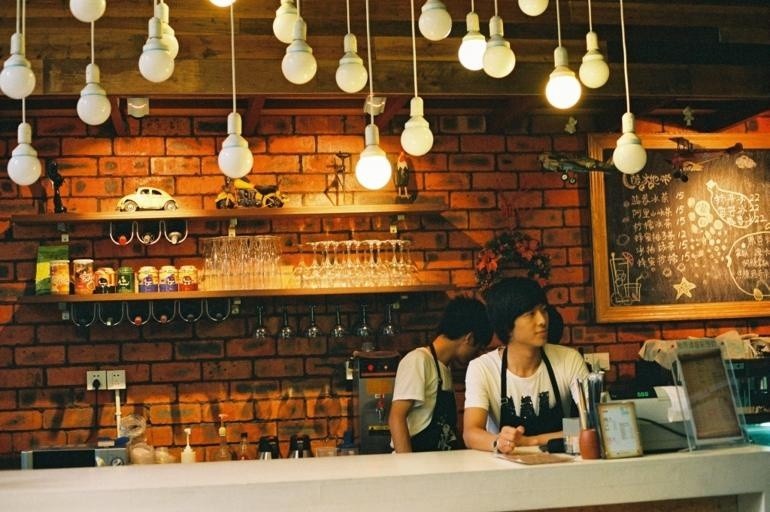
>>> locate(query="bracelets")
[493,439,502,454]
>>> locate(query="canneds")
[50,259,198,295]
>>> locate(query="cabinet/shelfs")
[10,203,458,304]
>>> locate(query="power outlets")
[106,369,127,390]
[594,352,610,371]
[581,352,594,372]
[87,371,106,391]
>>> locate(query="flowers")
[475,232,551,292]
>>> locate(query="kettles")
[257,434,314,460]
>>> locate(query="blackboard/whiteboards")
[587,132,770,323]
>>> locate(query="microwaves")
[21,447,128,469]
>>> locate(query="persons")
[387,294,494,454]
[462,275,590,455]
[393,152,408,196]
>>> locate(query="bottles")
[579,428,600,459]
[337,430,358,456]
[128,414,250,465]
[49,236,288,294]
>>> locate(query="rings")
[505,440,509,446]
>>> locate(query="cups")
[317,445,337,457]
[578,409,606,460]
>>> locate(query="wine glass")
[293,239,419,289]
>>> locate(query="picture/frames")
[594,401,643,460]
